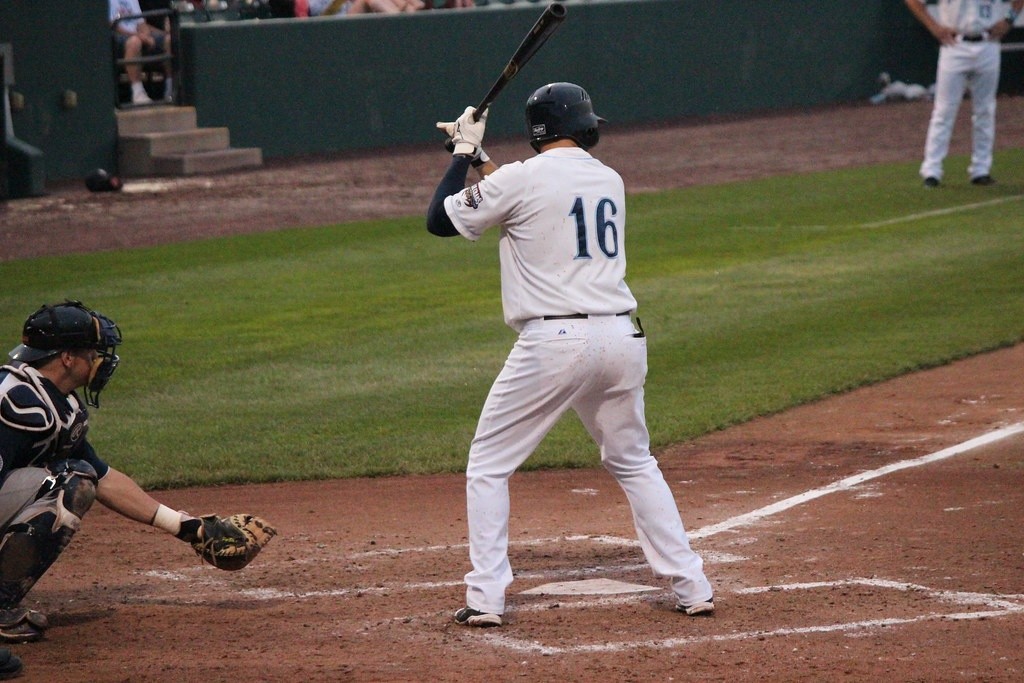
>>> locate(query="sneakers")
[454,606,503,626]
[676,596,715,615]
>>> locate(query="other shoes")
[0,648,23,680]
[134,92,152,106]
[0,607,48,642]
[971,176,997,187]
[925,177,939,188]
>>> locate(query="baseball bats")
[443,1,565,156]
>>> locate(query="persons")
[426,82,716,626]
[1,300,279,679]
[108,0,173,104]
[295,1,479,18]
[906,0,1023,188]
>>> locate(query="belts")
[963,35,983,43]
[545,311,630,319]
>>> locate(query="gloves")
[436,121,490,168]
[452,106,489,156]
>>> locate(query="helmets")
[526,82,608,153]
[6,302,122,409]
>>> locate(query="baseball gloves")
[189,509,280,575]
